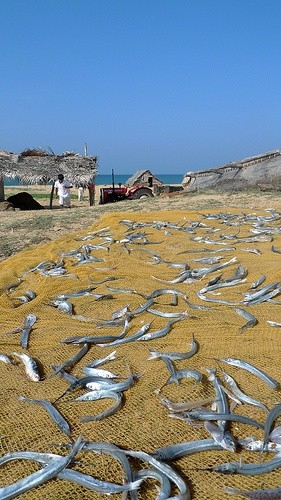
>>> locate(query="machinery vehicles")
[98,168,155,205]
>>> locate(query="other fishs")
[0,207,281,499]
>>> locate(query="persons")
[74,182,87,202]
[53,174,72,209]
